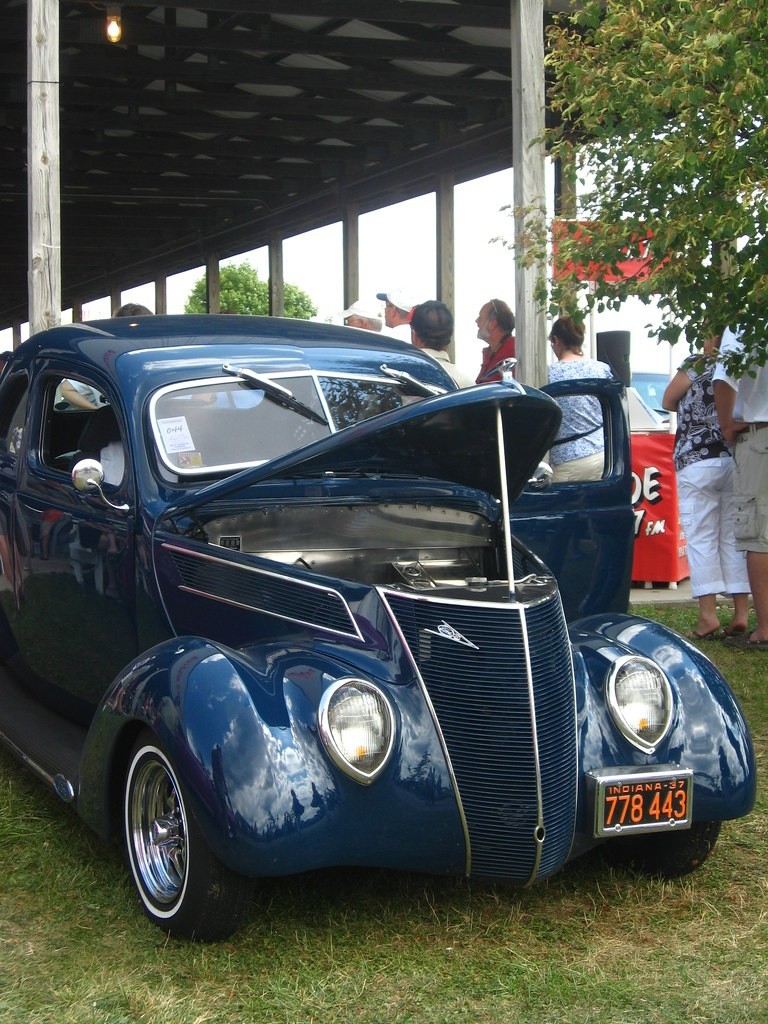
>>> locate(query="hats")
[343,299,384,320]
[408,299,455,342]
[376,276,419,313]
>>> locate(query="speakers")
[597,331,632,387]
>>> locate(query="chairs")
[72,405,126,485]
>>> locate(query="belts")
[739,422,768,433]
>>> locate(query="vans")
[623,355,672,432]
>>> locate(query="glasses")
[381,303,400,313]
[490,298,499,313]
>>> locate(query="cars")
[1,316,756,946]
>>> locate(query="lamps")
[107,6,122,42]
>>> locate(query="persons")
[339,288,468,406]
[477,299,515,383]
[665,315,768,652]
[59,303,213,412]
[35,509,121,601]
[544,314,611,481]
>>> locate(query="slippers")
[690,630,726,642]
[724,631,768,651]
